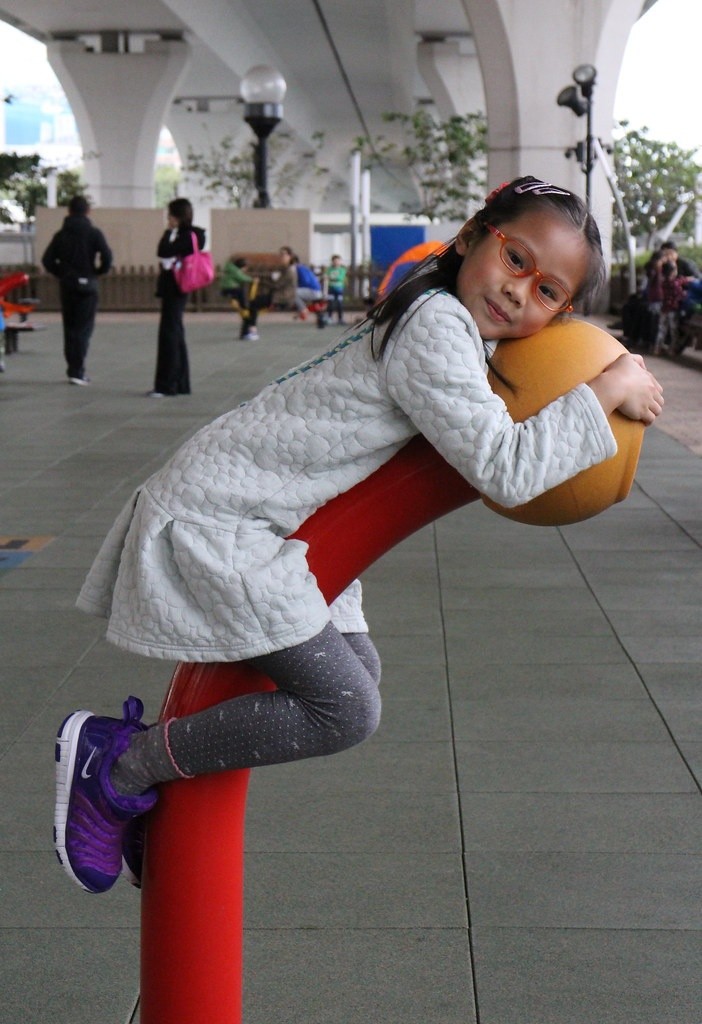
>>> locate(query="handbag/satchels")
[172,232,215,291]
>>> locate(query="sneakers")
[53,696,155,893]
[122,836,142,889]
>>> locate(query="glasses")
[483,223,575,315]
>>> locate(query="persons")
[292,255,322,321]
[248,248,298,332]
[324,255,345,325]
[607,241,702,354]
[147,199,206,397]
[53,176,665,894]
[222,258,259,341]
[41,196,111,386]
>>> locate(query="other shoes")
[605,322,624,332]
[244,331,260,341]
[147,391,172,397]
[71,377,90,385]
